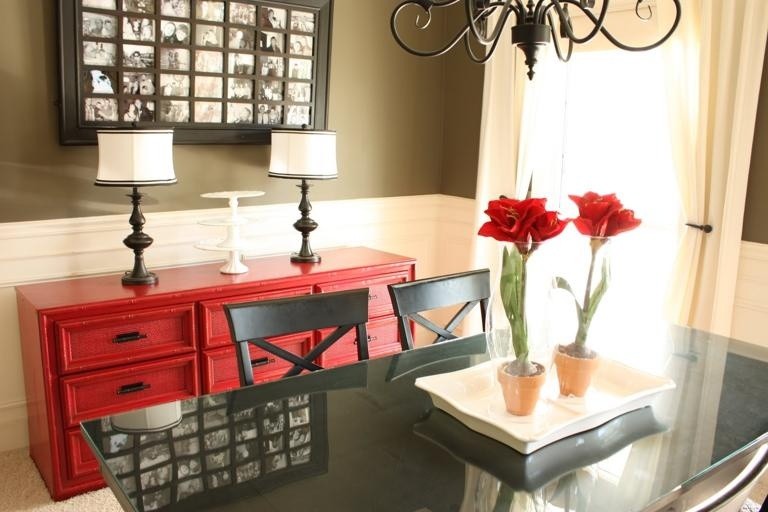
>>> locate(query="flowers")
[474,172,644,371]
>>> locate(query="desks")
[79,328,768,512]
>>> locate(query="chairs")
[222,269,495,388]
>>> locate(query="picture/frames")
[52,1,335,149]
[80,385,327,511]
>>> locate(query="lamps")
[91,129,339,288]
[390,0,683,86]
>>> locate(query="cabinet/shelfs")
[15,244,420,503]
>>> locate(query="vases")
[499,346,600,414]
